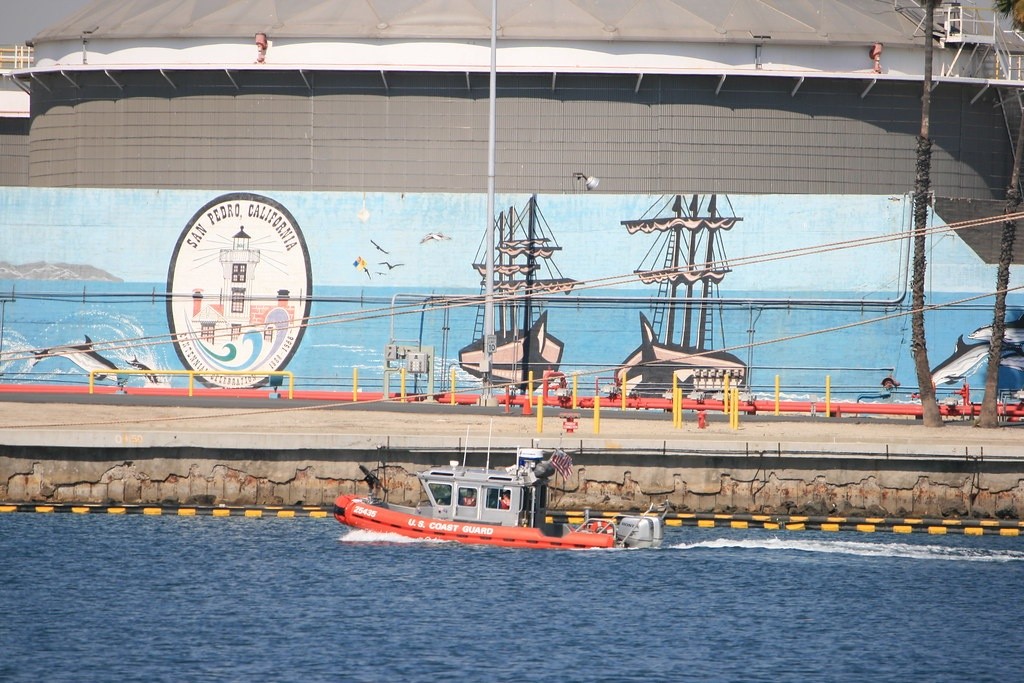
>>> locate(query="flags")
[550,450,572,482]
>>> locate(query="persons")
[500,492,509,509]
[463,489,475,506]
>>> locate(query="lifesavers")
[590,521,614,536]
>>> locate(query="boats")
[335,418,671,549]
